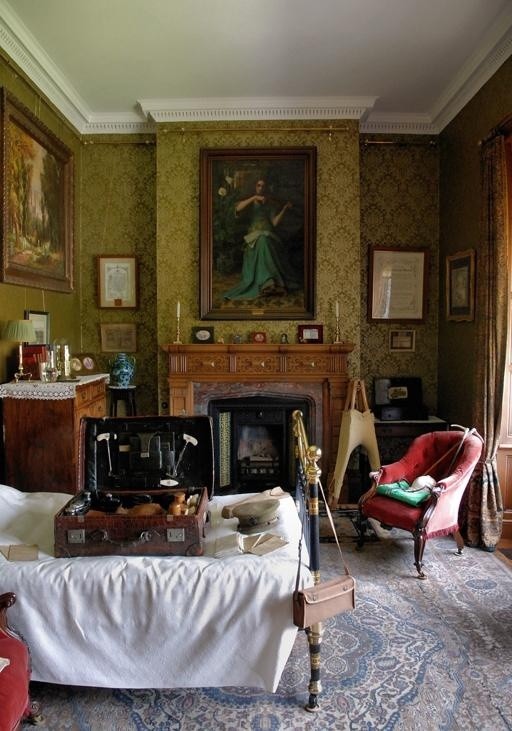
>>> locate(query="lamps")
[1,319,34,384]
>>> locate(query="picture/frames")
[445,249,474,322]
[100,255,140,310]
[368,247,429,325]
[100,324,136,354]
[389,329,416,353]
[191,328,215,344]
[0,88,76,294]
[298,324,323,344]
[200,147,318,323]
[26,309,50,346]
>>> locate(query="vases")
[110,350,137,388]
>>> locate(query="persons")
[223,178,298,300]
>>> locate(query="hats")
[234,500,282,535]
[409,475,436,502]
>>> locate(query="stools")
[107,385,139,416]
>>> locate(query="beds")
[1,411,322,715]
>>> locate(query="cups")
[217,336,224,344]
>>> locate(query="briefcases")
[53,414,215,558]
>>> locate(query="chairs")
[357,428,479,580]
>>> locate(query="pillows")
[380,476,432,505]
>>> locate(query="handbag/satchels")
[291,481,357,630]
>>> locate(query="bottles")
[106,353,136,387]
[66,492,198,516]
[281,333,287,345]
[38,345,74,383]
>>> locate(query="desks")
[374,409,449,437]
[1,374,110,492]
[159,345,355,503]
